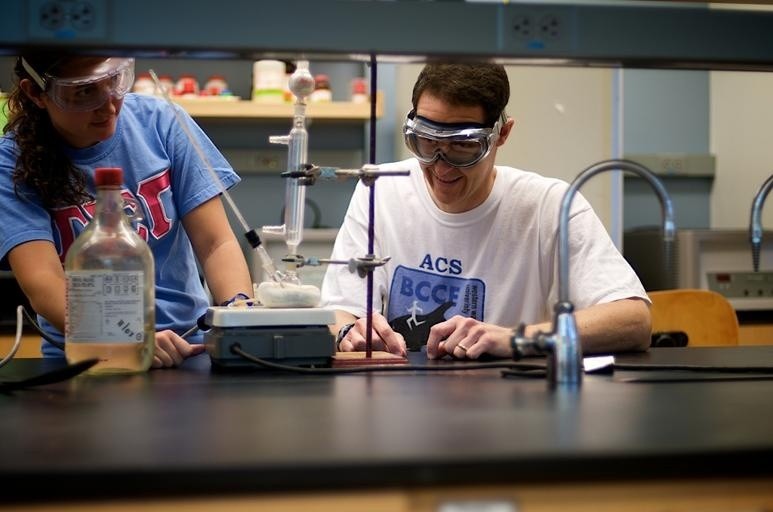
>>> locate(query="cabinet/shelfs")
[0,53,371,285]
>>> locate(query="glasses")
[20,56,137,114]
[401,104,509,169]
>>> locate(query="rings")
[458,344,467,351]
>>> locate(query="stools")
[618,286,741,355]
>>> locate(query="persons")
[318,64,652,363]
[0,57,254,369]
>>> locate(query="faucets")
[509,157,678,386]
[744,175,773,280]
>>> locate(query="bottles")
[63,167,155,378]
[350,79,367,105]
[313,74,332,104]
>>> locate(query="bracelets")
[337,323,355,352]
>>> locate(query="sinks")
[584,360,772,385]
